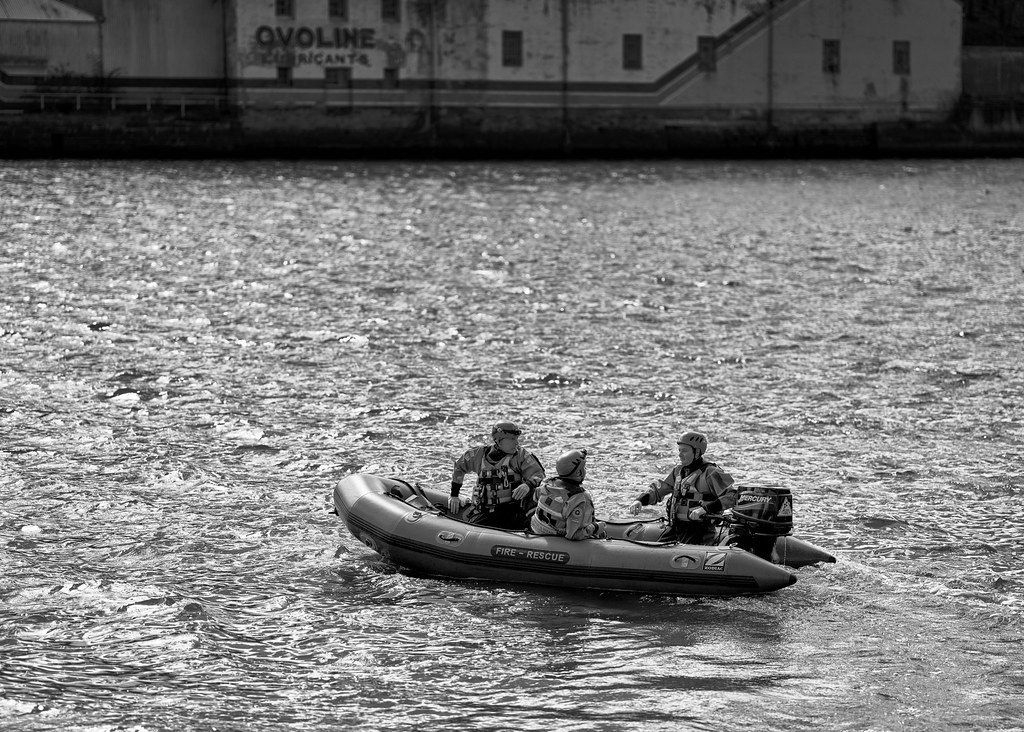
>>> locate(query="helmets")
[556,449,587,480]
[492,420,521,443]
[677,432,708,455]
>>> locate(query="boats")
[1,1,1023,160]
[333,471,836,595]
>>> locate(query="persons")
[448,420,547,530]
[629,431,739,545]
[526,448,608,541]
